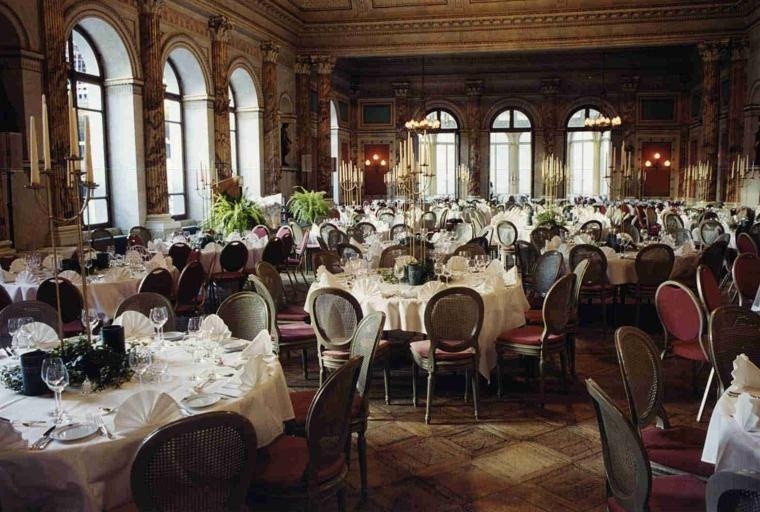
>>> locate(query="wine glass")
[8,319,22,354]
[651,231,673,246]
[434,263,444,281]
[41,358,68,418]
[82,308,99,344]
[560,229,595,245]
[459,251,490,277]
[640,229,647,247]
[323,217,353,230]
[149,309,165,349]
[46,366,69,427]
[18,317,36,351]
[340,253,367,287]
[188,317,205,353]
[183,335,198,365]
[442,265,451,285]
[201,328,219,361]
[153,306,168,347]
[394,263,405,283]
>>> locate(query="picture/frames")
[639,94,677,123]
[359,100,393,127]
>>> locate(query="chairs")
[575,219,603,245]
[521,249,564,311]
[208,241,249,314]
[352,213,368,223]
[287,219,314,276]
[190,252,218,317]
[389,223,415,241]
[246,353,365,512]
[661,211,695,243]
[467,236,490,256]
[452,242,487,275]
[493,271,578,410]
[584,375,709,512]
[353,221,377,244]
[696,261,723,323]
[425,209,448,240]
[731,251,760,308]
[284,310,387,503]
[419,210,437,232]
[703,466,760,511]
[698,218,724,247]
[254,259,311,361]
[409,285,485,424]
[514,239,541,298]
[319,222,339,251]
[477,225,494,253]
[710,231,731,249]
[112,290,177,333]
[168,241,192,271]
[379,211,397,228]
[261,235,299,301]
[653,279,715,421]
[286,228,312,289]
[496,220,526,270]
[312,250,345,280]
[35,276,86,339]
[308,286,395,406]
[726,231,760,304]
[523,256,591,377]
[129,225,152,248]
[91,228,115,251]
[0,299,62,356]
[615,221,641,251]
[568,243,619,335]
[129,410,259,511]
[248,273,316,380]
[525,248,564,307]
[317,235,333,255]
[176,258,206,316]
[274,224,294,253]
[137,266,175,299]
[406,239,435,263]
[696,240,726,288]
[644,205,661,241]
[336,242,365,262]
[619,243,676,328]
[378,243,416,269]
[250,224,271,241]
[216,290,272,342]
[530,227,553,259]
[708,302,760,402]
[125,244,152,262]
[328,228,351,254]
[0,285,11,311]
[718,246,737,295]
[614,324,715,485]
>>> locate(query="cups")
[129,348,151,391]
[146,348,168,390]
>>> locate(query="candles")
[40,93,52,173]
[454,161,473,185]
[84,114,95,184]
[601,138,648,186]
[538,153,566,187]
[26,113,41,187]
[69,105,82,158]
[382,128,439,187]
[335,156,364,188]
[681,157,715,182]
[729,152,756,184]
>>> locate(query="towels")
[241,329,273,359]
[485,209,524,234]
[227,231,239,241]
[149,252,168,269]
[239,359,274,388]
[313,264,332,283]
[471,278,493,292]
[104,266,129,278]
[172,234,187,243]
[58,269,84,283]
[446,254,470,272]
[13,271,38,285]
[481,258,504,275]
[419,280,448,299]
[720,352,759,402]
[351,280,379,299]
[198,312,232,342]
[111,311,155,340]
[395,254,417,269]
[10,322,59,352]
[113,390,182,429]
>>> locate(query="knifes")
[31,425,56,449]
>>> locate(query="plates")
[180,393,221,409]
[49,422,99,441]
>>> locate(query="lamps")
[645,152,673,168]
[583,49,623,136]
[363,153,388,173]
[404,56,442,133]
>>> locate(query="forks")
[94,416,111,441]
